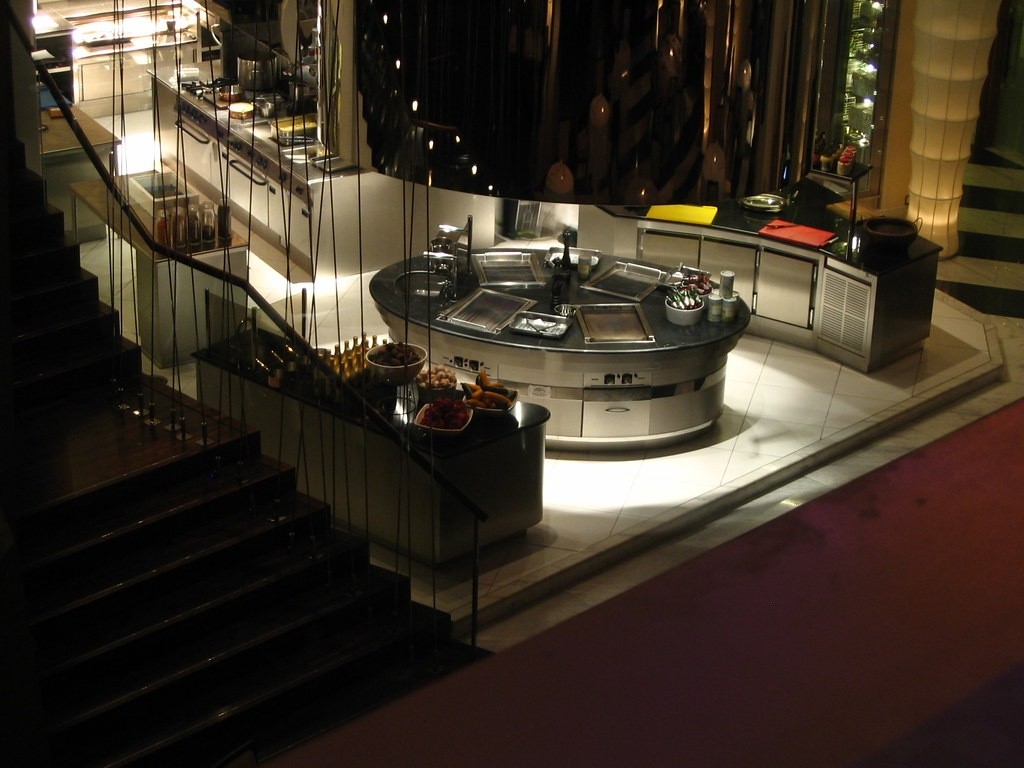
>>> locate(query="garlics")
[415,364,456,388]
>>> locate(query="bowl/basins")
[419,382,458,405]
[664,299,704,326]
[365,341,428,385]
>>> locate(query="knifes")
[821,237,841,249]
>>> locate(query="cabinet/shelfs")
[76,42,202,118]
[641,231,819,331]
[34,34,73,104]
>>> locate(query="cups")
[578,254,591,281]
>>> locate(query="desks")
[67,169,250,369]
[40,105,122,244]
[190,327,551,574]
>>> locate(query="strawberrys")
[420,398,469,430]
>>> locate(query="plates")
[463,388,519,418]
[414,403,474,436]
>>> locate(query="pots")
[236,51,279,91]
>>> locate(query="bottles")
[267,333,388,409]
[817,142,857,175]
[186,201,202,247]
[664,284,702,310]
[201,200,215,244]
[157,210,170,254]
[216,197,233,241]
[707,269,741,322]
[172,206,186,249]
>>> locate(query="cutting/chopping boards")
[759,219,836,248]
[646,205,718,226]
